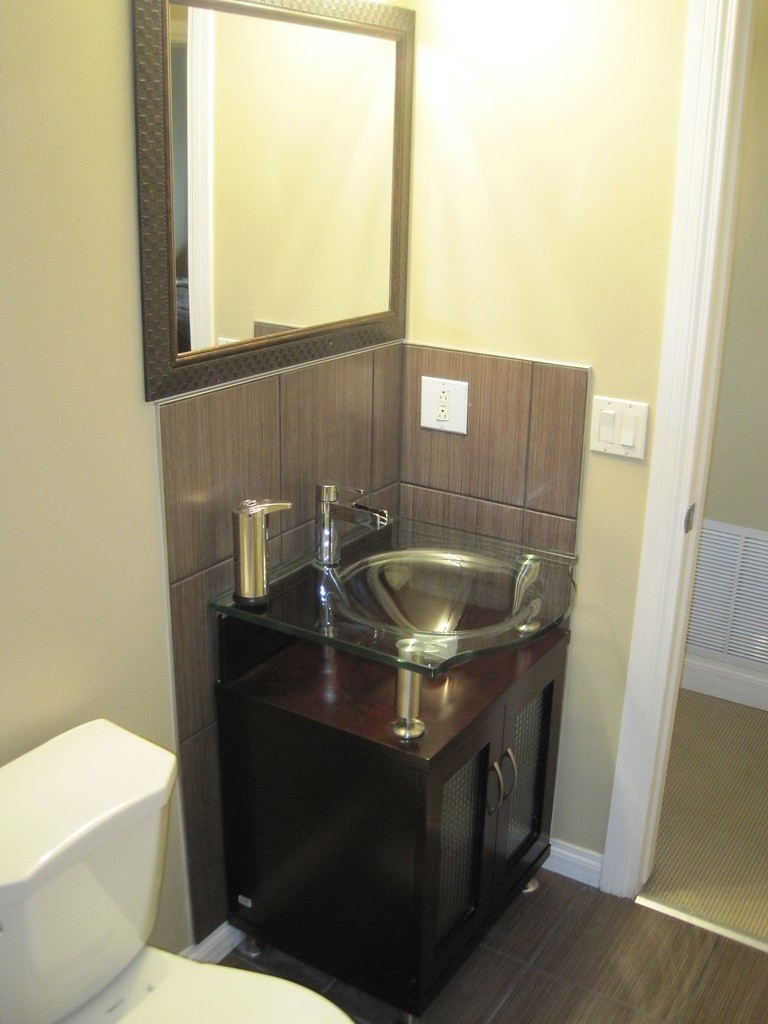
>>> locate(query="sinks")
[331,546,544,639]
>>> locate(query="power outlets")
[420,376,467,435]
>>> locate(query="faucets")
[313,479,392,562]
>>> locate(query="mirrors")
[127,0,418,402]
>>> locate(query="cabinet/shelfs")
[217,587,570,1024]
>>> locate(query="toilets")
[0,718,356,1024]
